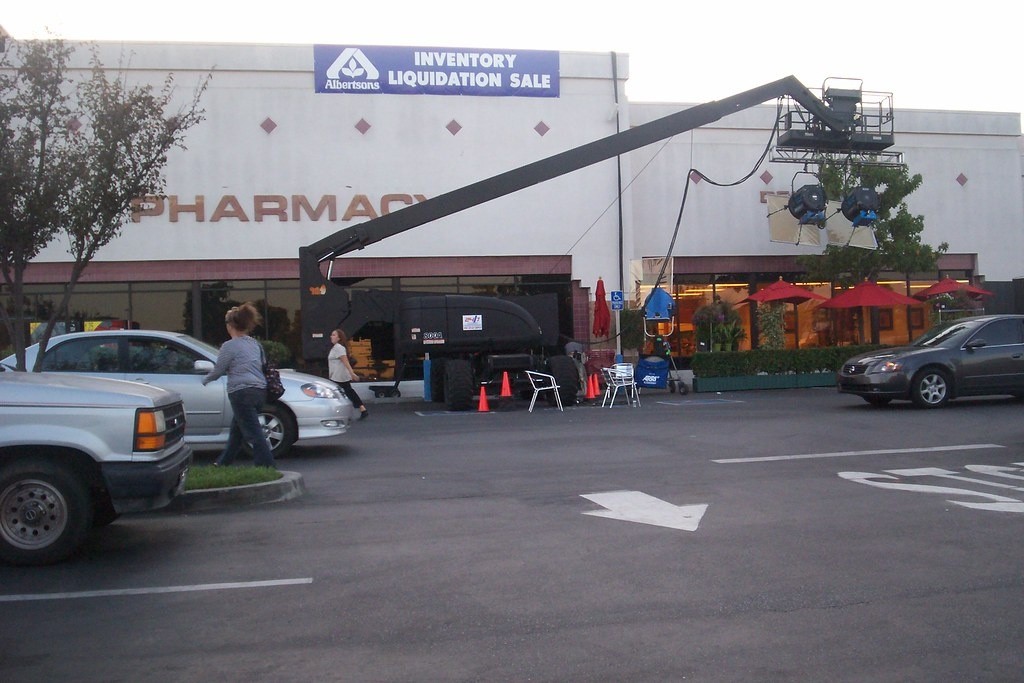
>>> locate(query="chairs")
[601,363,641,408]
[524,371,563,413]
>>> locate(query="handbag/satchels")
[633,358,672,389]
[348,357,357,366]
[258,344,285,405]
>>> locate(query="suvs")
[0,366,193,568]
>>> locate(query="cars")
[835,314,1023,412]
[0,328,353,460]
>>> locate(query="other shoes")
[357,410,369,421]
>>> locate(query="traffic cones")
[499,370,513,398]
[584,375,597,399]
[591,373,601,396]
[477,386,491,413]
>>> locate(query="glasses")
[225,320,231,324]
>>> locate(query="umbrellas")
[593,276,610,351]
[912,274,994,300]
[734,275,828,331]
[815,277,923,344]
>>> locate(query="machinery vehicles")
[298,72,907,413]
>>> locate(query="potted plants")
[712,323,726,351]
[723,319,743,352]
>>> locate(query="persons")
[201,304,276,469]
[327,329,369,421]
[158,349,181,374]
